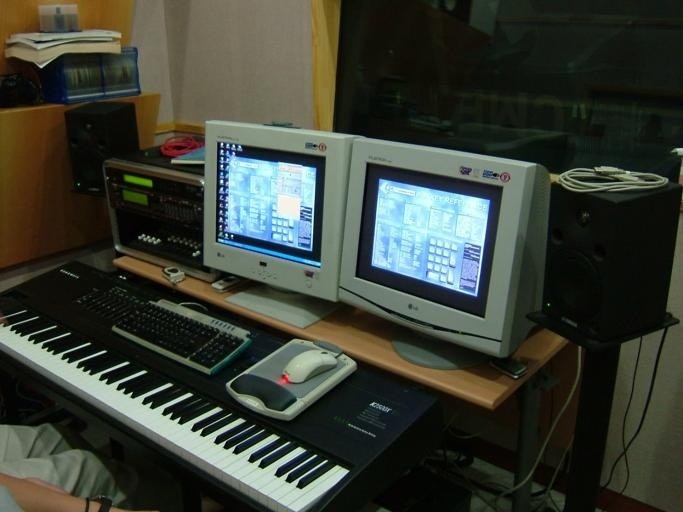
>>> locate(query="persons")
[0,417,163,512]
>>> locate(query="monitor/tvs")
[338,137,552,369]
[205,121,355,329]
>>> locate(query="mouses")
[281,345,338,385]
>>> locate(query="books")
[5,29,124,71]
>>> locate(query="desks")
[112,254,569,511]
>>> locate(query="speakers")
[542,175,683,340]
[68,103,137,197]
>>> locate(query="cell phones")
[487,357,529,378]
[212,275,238,292]
[163,265,187,285]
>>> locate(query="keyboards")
[110,299,254,375]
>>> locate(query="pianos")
[0,259,442,512]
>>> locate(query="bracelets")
[85,497,89,512]
[92,495,112,512]
